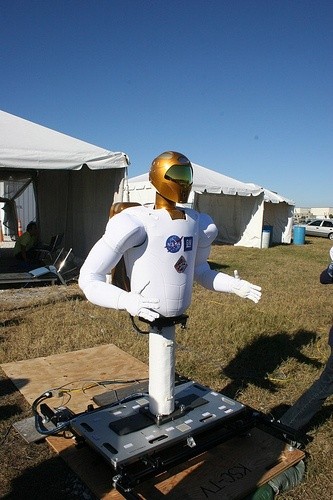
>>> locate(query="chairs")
[35,233,59,266]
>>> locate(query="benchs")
[0,247,79,286]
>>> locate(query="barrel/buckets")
[262,225,273,246]
[293,225,305,245]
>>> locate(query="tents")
[246,183,295,243]
[112,161,264,248]
[0,109,130,287]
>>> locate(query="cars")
[292,218,333,240]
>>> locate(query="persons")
[12,221,37,260]
[78,151,263,321]
[278,247,333,431]
